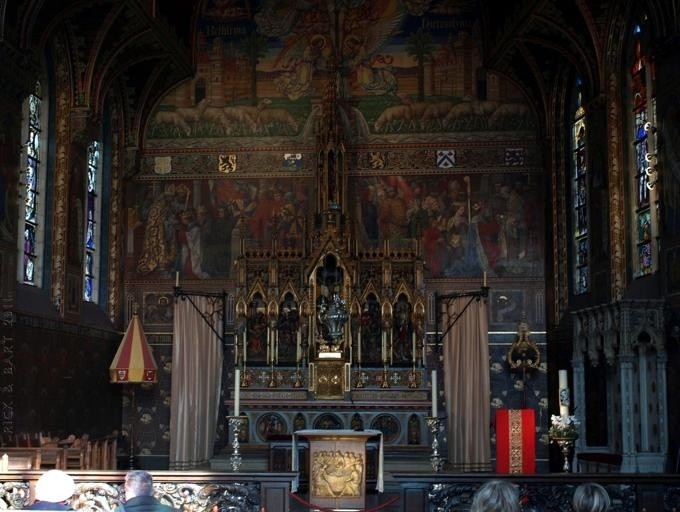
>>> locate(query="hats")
[34,469,74,503]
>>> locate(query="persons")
[111,469,182,511]
[312,450,363,497]
[470,480,522,512]
[573,482,610,512]
[17,469,80,510]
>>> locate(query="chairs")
[0,428,119,471]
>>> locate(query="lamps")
[108,301,158,469]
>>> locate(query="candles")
[233,368,241,416]
[431,370,441,417]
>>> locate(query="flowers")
[549,415,580,438]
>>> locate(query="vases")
[548,435,579,473]
